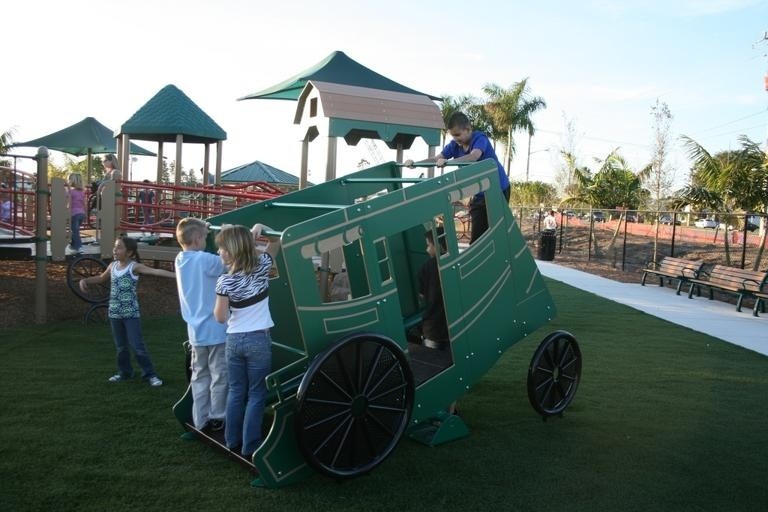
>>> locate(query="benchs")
[641,256,704,297]
[688,264,768,312]
[751,292,768,317]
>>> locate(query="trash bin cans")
[538,229,556,261]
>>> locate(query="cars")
[659,215,682,225]
[528,207,608,223]
[621,214,634,223]
[633,216,643,223]
[694,218,716,229]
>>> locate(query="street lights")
[524,147,549,182]
[128,156,137,199]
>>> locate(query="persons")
[201,167,213,185]
[100,153,121,188]
[80,237,177,386]
[404,112,512,246]
[418,228,450,351]
[212,223,279,456]
[135,180,155,223]
[174,216,233,432]
[65,174,85,252]
[543,210,559,229]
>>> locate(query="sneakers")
[210,420,224,432]
[109,375,126,382]
[149,377,162,387]
